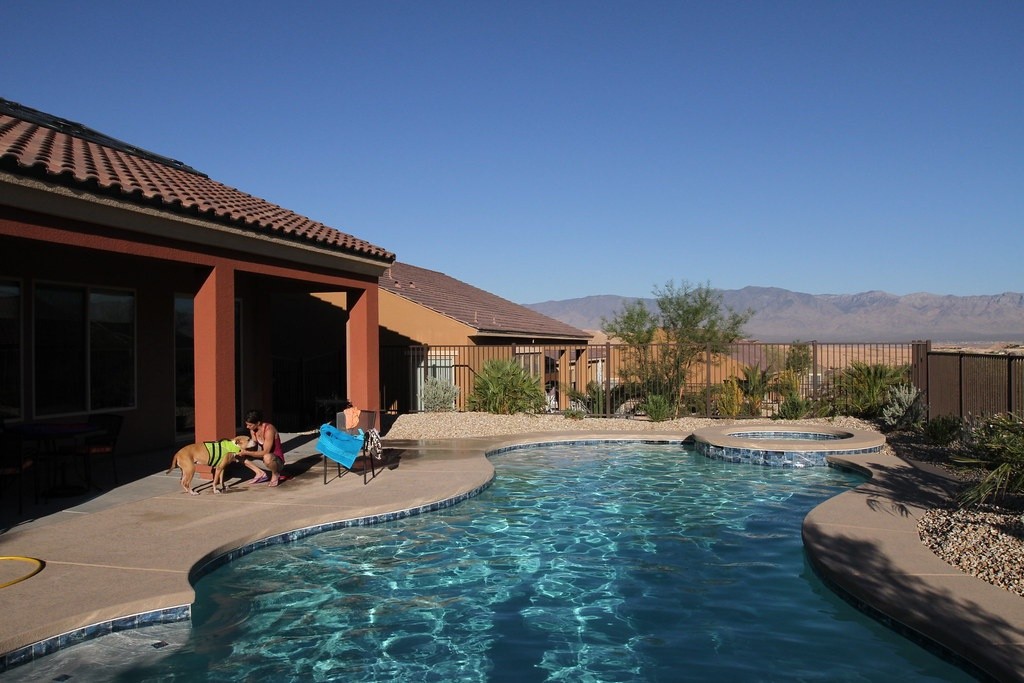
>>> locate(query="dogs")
[165,435,257,496]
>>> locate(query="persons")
[236,411,285,486]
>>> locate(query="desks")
[3,421,107,513]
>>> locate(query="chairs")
[323,410,377,485]
[0,411,125,515]
